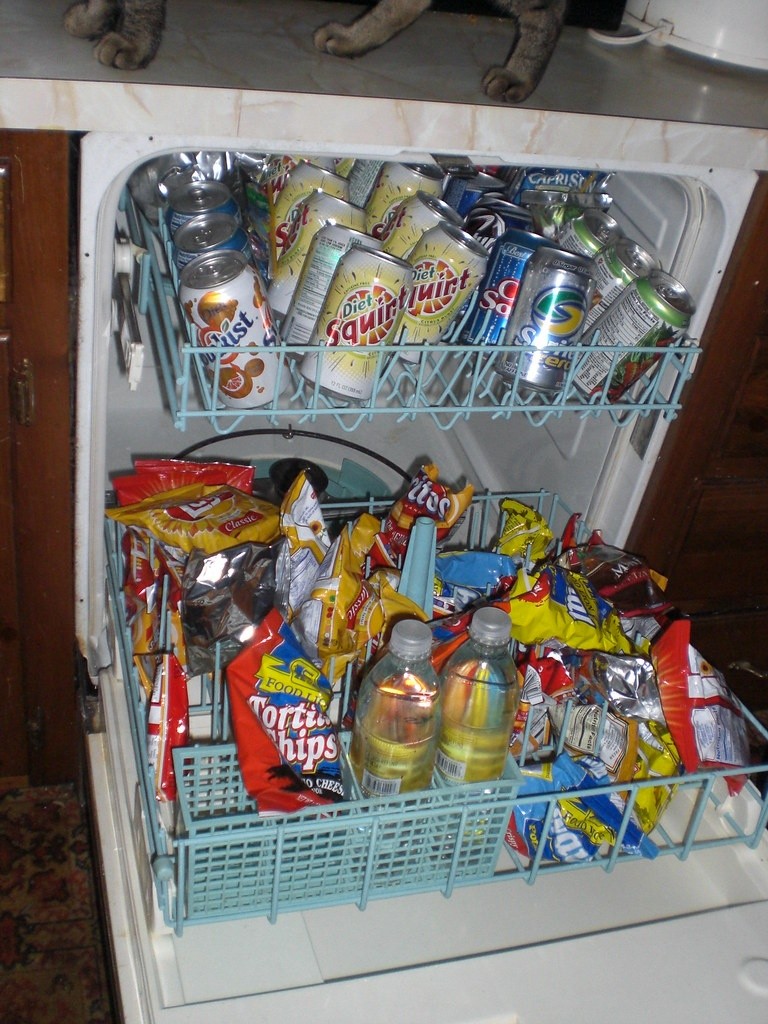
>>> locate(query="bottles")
[348,620,442,877]
[440,607,520,853]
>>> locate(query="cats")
[63,0,568,105]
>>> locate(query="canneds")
[165,154,696,413]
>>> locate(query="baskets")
[172,729,527,919]
